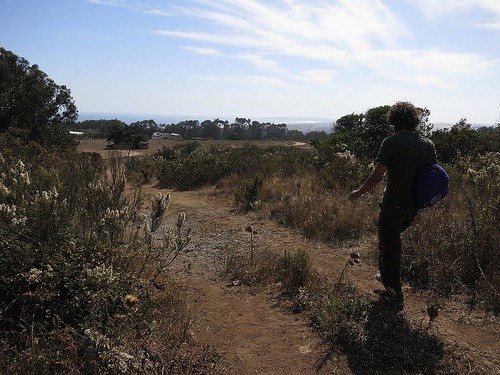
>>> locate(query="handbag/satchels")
[414,163,450,209]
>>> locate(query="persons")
[348,101,438,310]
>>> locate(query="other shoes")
[375,273,405,309]
